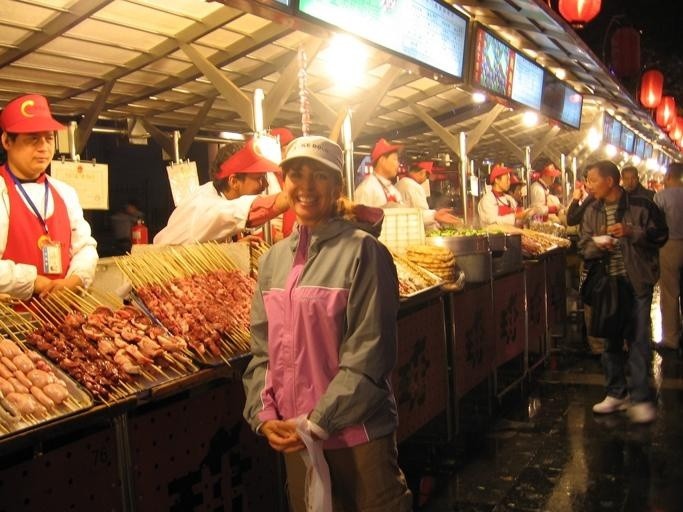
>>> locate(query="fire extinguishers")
[130,217,149,245]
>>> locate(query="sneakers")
[629,402,655,423]
[593,392,630,414]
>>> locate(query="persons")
[620,167,654,201]
[653,161,683,353]
[152,139,290,244]
[270,128,300,244]
[506,175,525,201]
[353,138,463,227]
[577,160,668,424]
[566,164,596,226]
[575,167,588,207]
[476,166,539,227]
[393,159,433,210]
[0,93,100,301]
[530,163,562,221]
[241,136,415,512]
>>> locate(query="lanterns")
[610,26,641,80]
[669,117,682,140]
[676,136,683,151]
[664,108,676,132]
[655,96,675,128]
[639,70,663,109]
[558,0,602,30]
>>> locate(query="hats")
[510,176,527,187]
[539,163,561,178]
[411,159,434,179]
[490,164,513,184]
[371,138,403,165]
[269,128,295,150]
[0,93,65,133]
[215,139,279,180]
[279,135,344,175]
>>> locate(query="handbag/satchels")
[294,414,332,512]
[579,261,607,302]
